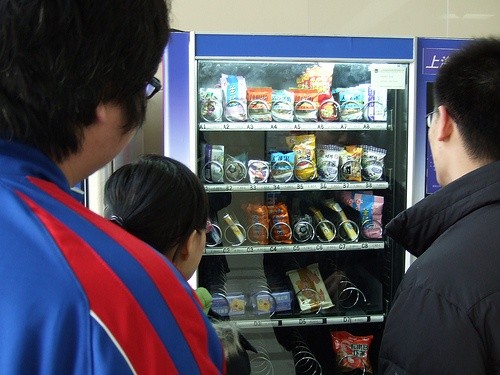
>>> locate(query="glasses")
[425,106,459,127]
[143,76,161,100]
[206,220,213,234]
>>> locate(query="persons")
[198,129,258,322]
[263,131,386,375]
[103,155,253,375]
[0,0,225,375]
[378,38,500,375]
